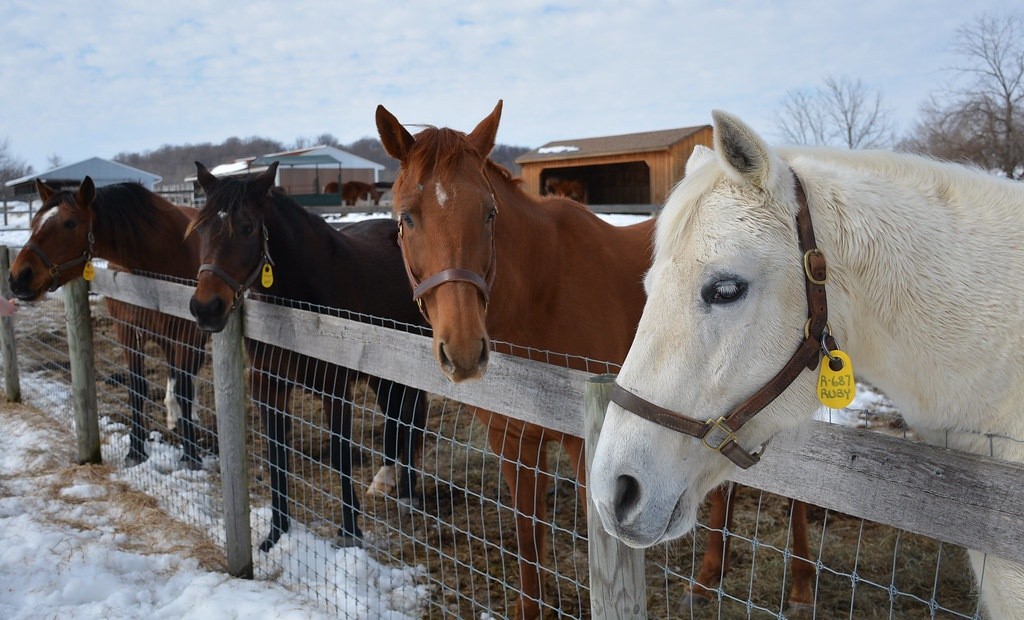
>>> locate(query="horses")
[188,158,436,553]
[7,175,214,472]
[590,107,1024,620]
[376,101,825,620]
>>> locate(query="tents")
[3,157,163,230]
[183,144,387,223]
[251,154,343,205]
